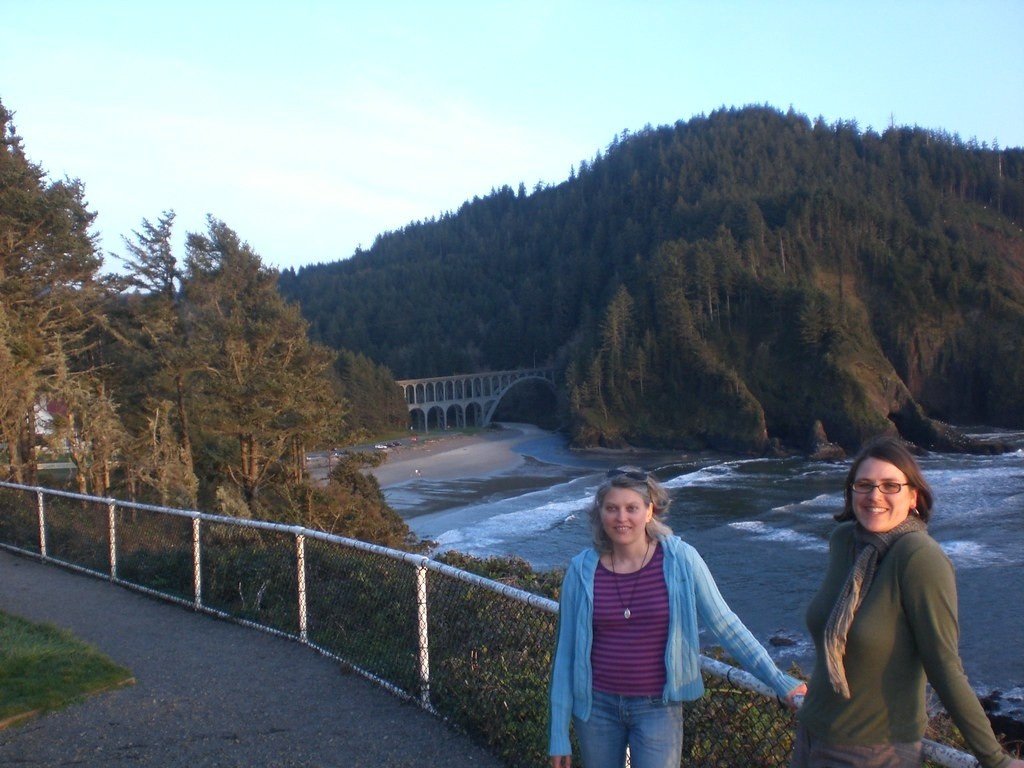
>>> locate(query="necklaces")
[611,541,649,618]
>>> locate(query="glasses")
[850,482,910,494]
[605,469,649,488]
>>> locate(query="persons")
[788,439,1024,768]
[549,465,807,768]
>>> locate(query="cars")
[375,441,402,450]
